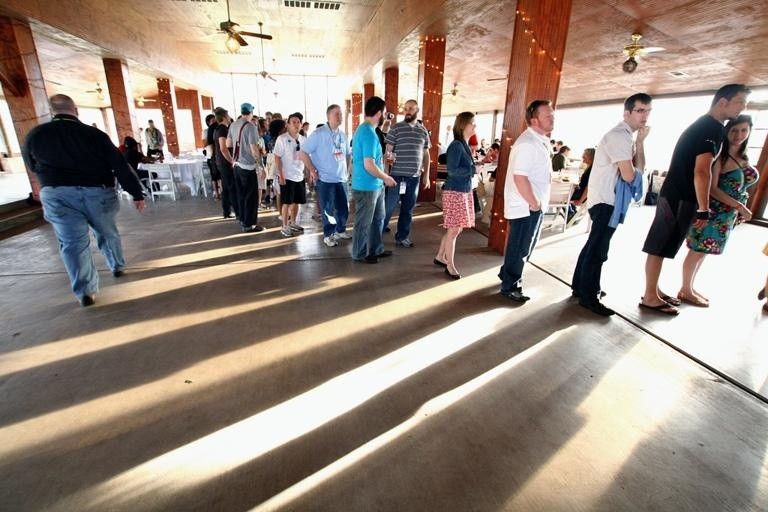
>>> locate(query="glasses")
[296,140,300,151]
[631,107,650,113]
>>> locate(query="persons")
[350,96,432,262]
[638,85,751,318]
[498,99,555,303]
[758,243,768,313]
[550,139,596,226]
[681,114,761,307]
[434,111,500,280]
[203,103,355,246]
[116,120,164,197]
[572,94,653,316]
[21,91,150,309]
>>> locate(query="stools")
[113,175,135,201]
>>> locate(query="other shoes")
[402,239,413,246]
[445,266,461,279]
[312,215,321,222]
[579,300,615,316]
[500,289,528,302]
[364,256,378,264]
[247,225,264,232]
[434,258,447,267]
[385,227,390,232]
[677,288,708,306]
[378,251,392,258]
[572,288,605,298]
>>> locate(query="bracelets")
[696,208,712,218]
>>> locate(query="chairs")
[646,171,667,207]
[549,182,572,233]
[149,169,177,202]
[472,166,496,214]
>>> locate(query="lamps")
[221,37,240,57]
[623,56,638,72]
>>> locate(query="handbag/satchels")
[231,144,240,161]
[645,191,658,205]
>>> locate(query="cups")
[386,151,396,165]
[553,172,571,183]
[385,112,394,122]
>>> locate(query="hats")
[241,103,254,115]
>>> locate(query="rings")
[143,204,147,208]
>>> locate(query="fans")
[623,33,666,57]
[83,80,157,108]
[251,22,277,82]
[442,83,466,99]
[187,1,271,46]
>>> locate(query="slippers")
[639,302,679,315]
[641,294,680,306]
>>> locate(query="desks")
[136,149,210,198]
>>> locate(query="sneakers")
[113,270,125,277]
[335,231,352,239]
[323,236,338,247]
[280,225,293,236]
[290,223,303,232]
[80,293,95,307]
